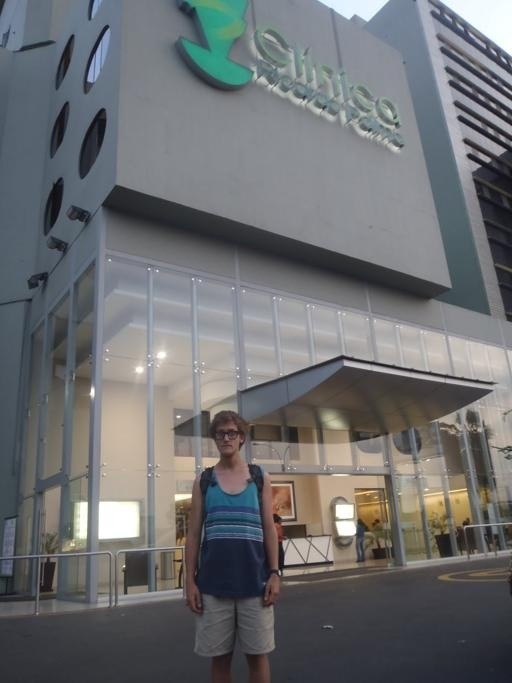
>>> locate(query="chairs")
[122,547,158,595]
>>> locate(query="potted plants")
[425,510,455,556]
[365,520,393,559]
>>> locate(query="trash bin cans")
[161,551,175,580]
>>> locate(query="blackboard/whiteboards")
[0,515,18,578]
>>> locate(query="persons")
[354,518,365,563]
[273,513,284,570]
[183,411,282,683]
[372,519,382,530]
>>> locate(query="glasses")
[213,430,242,442]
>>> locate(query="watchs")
[269,569,282,576]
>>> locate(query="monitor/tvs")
[334,502,356,522]
[332,522,358,539]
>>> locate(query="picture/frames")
[271,478,297,521]
